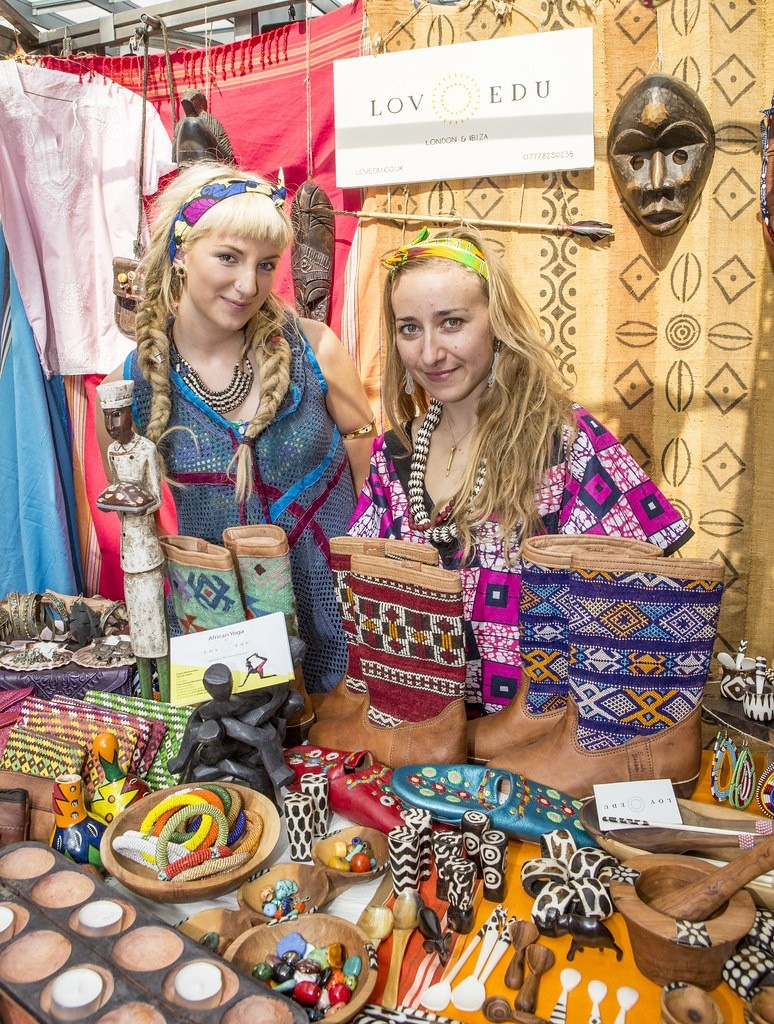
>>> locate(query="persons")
[91,162,380,693]
[348,225,694,560]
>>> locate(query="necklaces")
[442,403,481,473]
[406,397,490,546]
[162,321,253,413]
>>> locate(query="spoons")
[514,945,555,1013]
[550,968,581,1024]
[614,986,639,1024]
[420,904,517,1012]
[587,979,608,1024]
[504,921,539,989]
[382,888,426,1011]
[483,997,553,1024]
[358,905,394,955]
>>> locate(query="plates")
[72,645,137,668]
[0,650,73,671]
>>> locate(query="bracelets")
[117,783,263,882]
[0,592,127,637]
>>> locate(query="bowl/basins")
[744,986,774,1024]
[178,909,252,956]
[659,982,724,1024]
[238,863,328,922]
[99,783,281,903]
[222,912,378,1024]
[311,825,391,884]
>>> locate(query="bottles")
[91,733,153,824]
[49,773,109,872]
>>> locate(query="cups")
[721,663,756,701]
[742,683,774,721]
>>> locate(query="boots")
[487,550,725,806]
[158,535,246,634]
[467,535,663,763]
[220,525,315,750]
[303,537,440,722]
[307,553,466,774]
